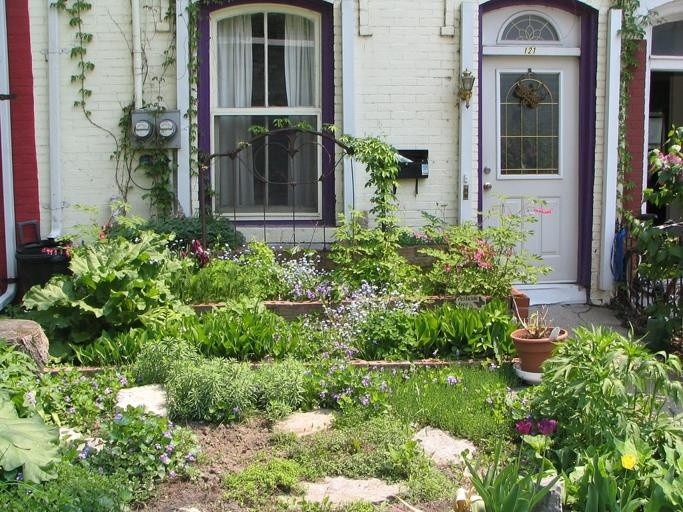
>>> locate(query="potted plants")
[511,295,569,385]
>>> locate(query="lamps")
[455,66,475,109]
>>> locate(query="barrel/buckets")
[15,238,71,300]
